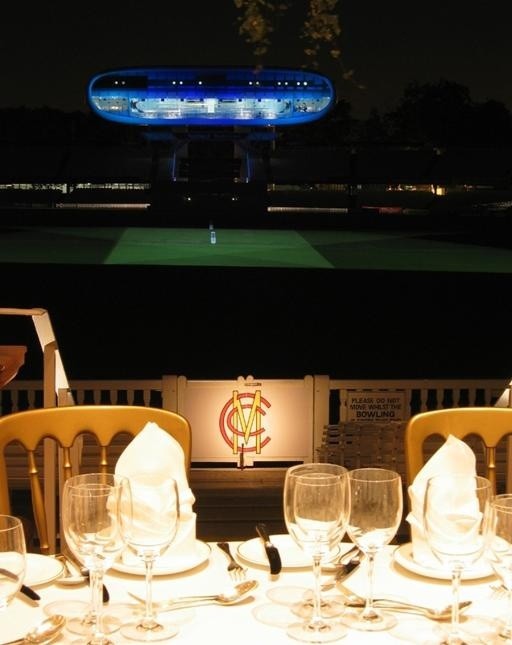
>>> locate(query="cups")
[0,515,27,613]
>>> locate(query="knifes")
[254,522,282,575]
[0,567,41,603]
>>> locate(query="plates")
[23,552,66,589]
[111,539,212,578]
[237,533,341,569]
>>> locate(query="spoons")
[1,614,68,645]
[152,578,259,609]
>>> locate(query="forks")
[216,541,246,584]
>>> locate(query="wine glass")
[285,461,512,644]
[60,472,179,645]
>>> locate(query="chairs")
[404,405,510,549]
[2,405,192,566]
